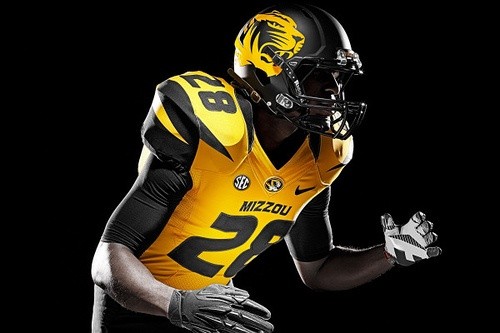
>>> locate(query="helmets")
[228,4,368,140]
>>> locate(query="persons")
[91,4,442,333]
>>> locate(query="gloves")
[168,284,274,333]
[381,212,442,266]
